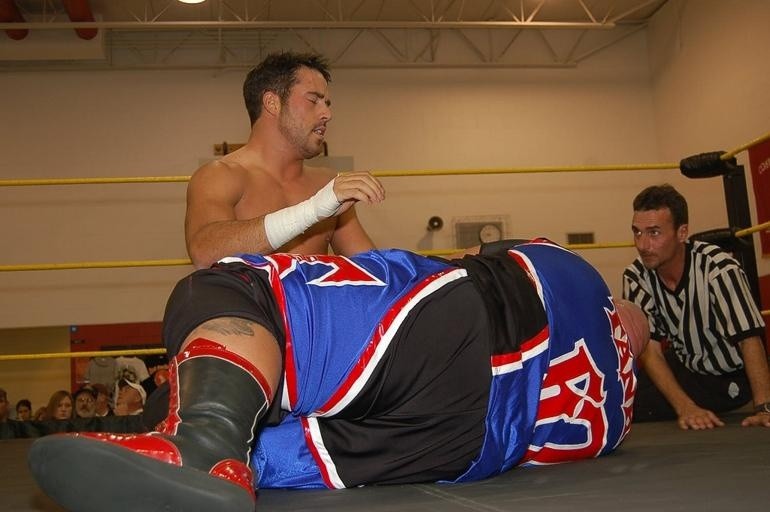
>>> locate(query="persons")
[21,236,653,511]
[181,46,389,272]
[622,183,770,431]
[0,355,168,439]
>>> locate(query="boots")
[27,337,275,512]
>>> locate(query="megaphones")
[427,215,444,231]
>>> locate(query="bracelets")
[755,401,770,413]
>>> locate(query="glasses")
[75,396,95,404]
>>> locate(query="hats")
[77,383,109,398]
[118,377,148,407]
